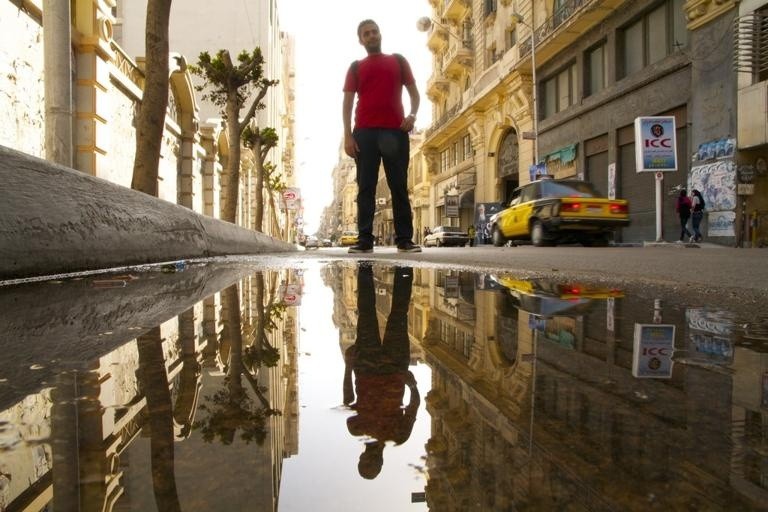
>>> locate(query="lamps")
[417,16,466,46]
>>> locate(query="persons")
[676,190,694,243]
[341,18,423,254]
[690,190,705,242]
[342,263,421,479]
[477,203,498,244]
[375,225,476,247]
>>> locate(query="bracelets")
[409,381,417,388]
[408,113,417,121]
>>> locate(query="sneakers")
[347,243,375,254]
[397,242,421,253]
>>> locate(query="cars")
[337,230,359,247]
[305,237,318,248]
[424,226,468,247]
[323,238,332,247]
[489,179,629,247]
[485,274,625,319]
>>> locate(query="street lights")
[510,12,539,165]
